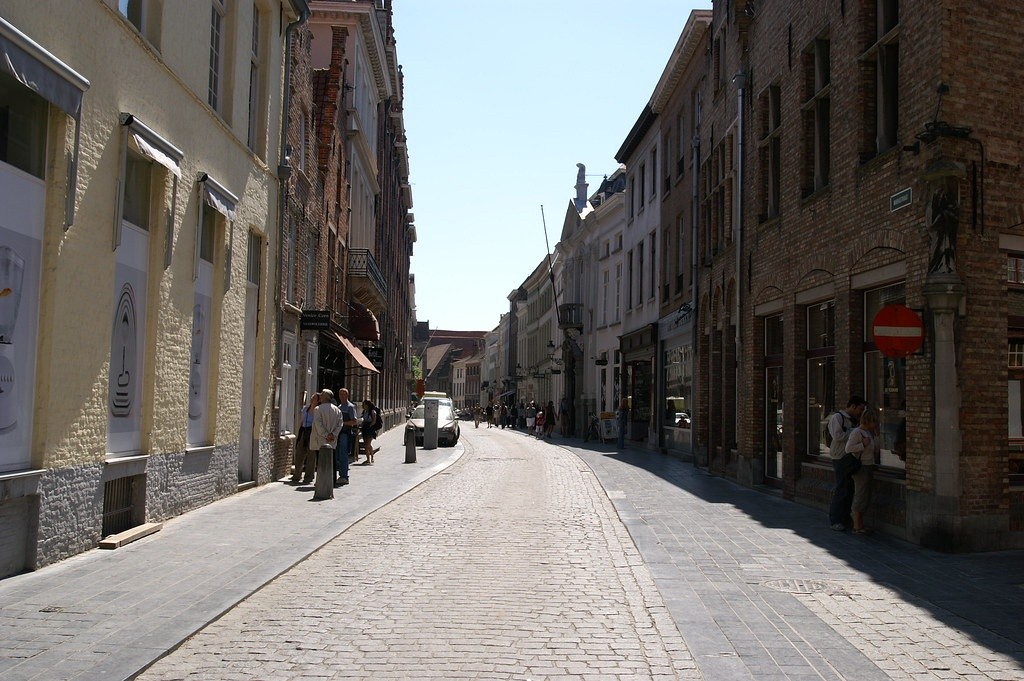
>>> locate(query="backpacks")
[823,411,845,449]
[369,402,383,431]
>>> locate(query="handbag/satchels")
[844,453,863,475]
[562,410,568,415]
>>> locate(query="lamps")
[546,340,561,361]
[493,379,497,388]
[516,363,521,373]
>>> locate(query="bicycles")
[584,412,611,444]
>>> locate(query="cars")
[414,391,458,416]
[403,404,461,447]
[675,412,690,428]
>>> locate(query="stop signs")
[871,301,926,359]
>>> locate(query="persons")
[665,400,691,428]
[845,409,881,536]
[408,400,418,416]
[473,398,572,439]
[288,388,376,487]
[827,396,865,531]
[616,398,629,450]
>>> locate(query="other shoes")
[303,478,312,482]
[336,477,349,484]
[852,526,871,535]
[831,523,845,531]
[362,461,370,466]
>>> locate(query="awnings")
[326,302,382,375]
